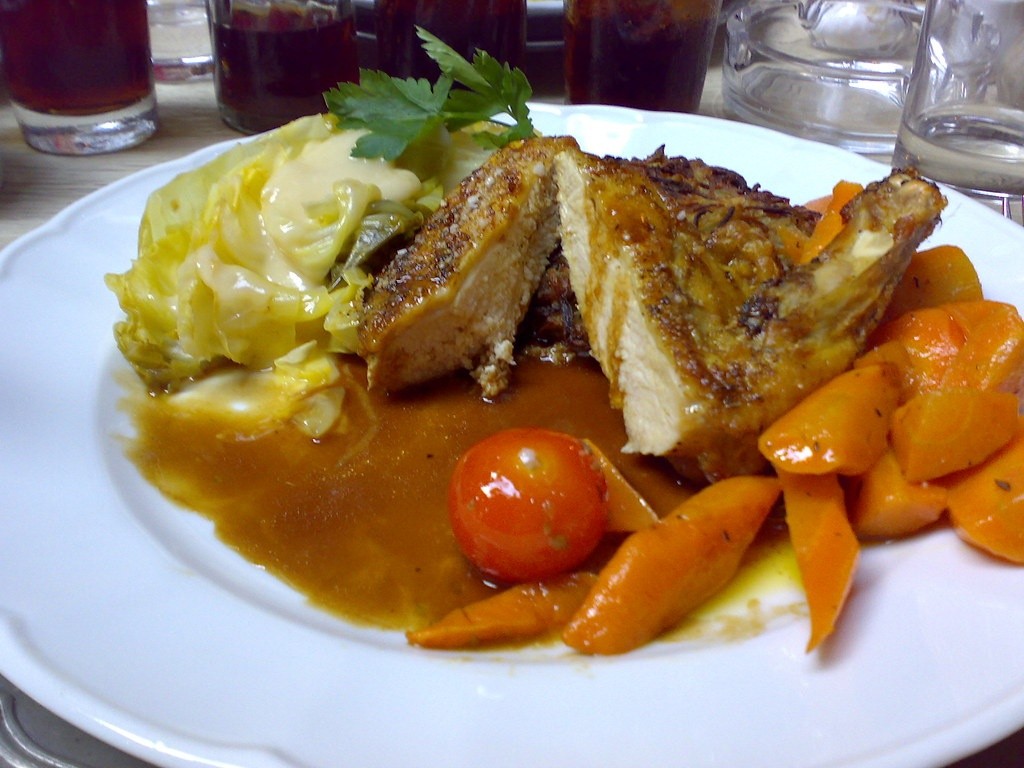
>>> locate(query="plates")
[1,102,1024,768]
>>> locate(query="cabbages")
[102,106,547,442]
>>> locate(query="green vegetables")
[319,26,541,160]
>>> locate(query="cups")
[558,0,726,114]
[373,0,529,92]
[890,0,1024,200]
[146,1,213,81]
[723,1,925,154]
[205,0,361,135]
[0,0,157,156]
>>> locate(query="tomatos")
[445,426,609,583]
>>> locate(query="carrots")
[405,179,1024,657]
